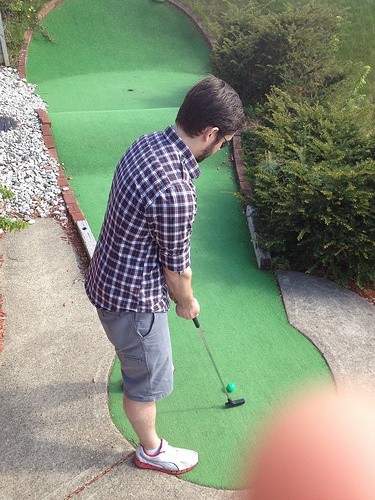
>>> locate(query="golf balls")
[226,382,236,393]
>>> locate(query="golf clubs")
[193,317,246,408]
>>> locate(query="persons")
[82,75,247,476]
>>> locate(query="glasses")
[213,126,231,149]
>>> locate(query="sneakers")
[134,438,200,475]
